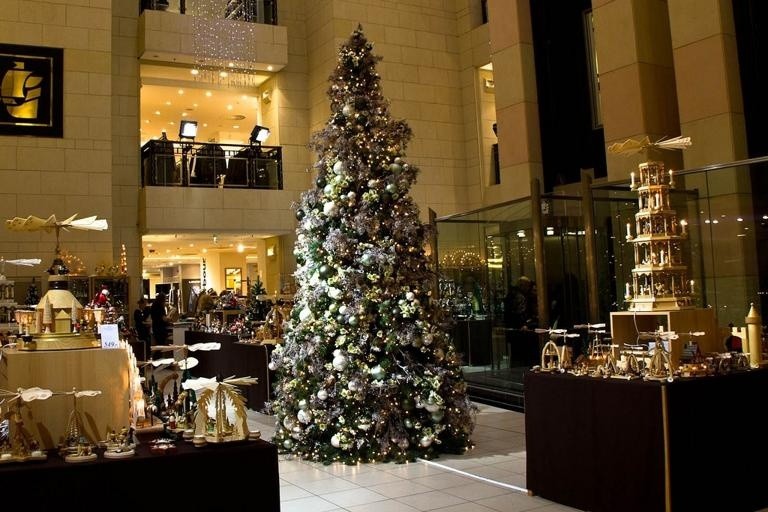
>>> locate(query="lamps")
[179,120,198,143]
[249,125,271,146]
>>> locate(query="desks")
[524,312,767,509]
[0,309,298,512]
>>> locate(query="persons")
[134,298,152,355]
[504,276,534,328]
[150,293,176,357]
